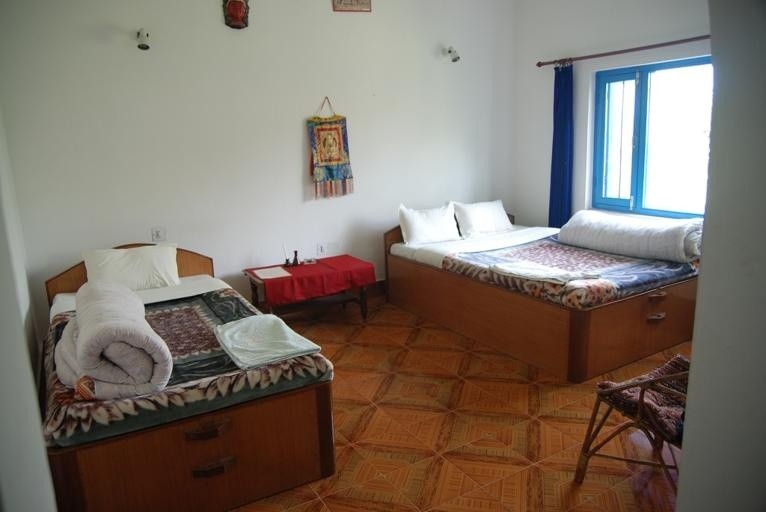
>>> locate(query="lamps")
[136,28,150,51]
[442,46,460,63]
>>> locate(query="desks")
[241,255,377,323]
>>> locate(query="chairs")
[574,352,691,495]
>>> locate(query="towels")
[212,313,321,370]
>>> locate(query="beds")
[383,212,700,383]
[35,242,336,512]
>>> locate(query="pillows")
[81,245,179,292]
[398,199,515,246]
